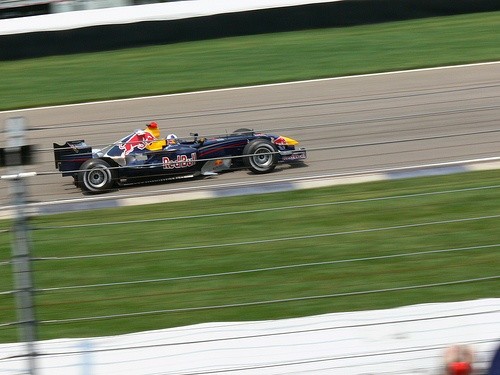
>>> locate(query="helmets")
[166,134,178,145]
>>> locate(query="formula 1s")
[52,127,307,196]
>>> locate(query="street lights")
[0,115,52,342]
